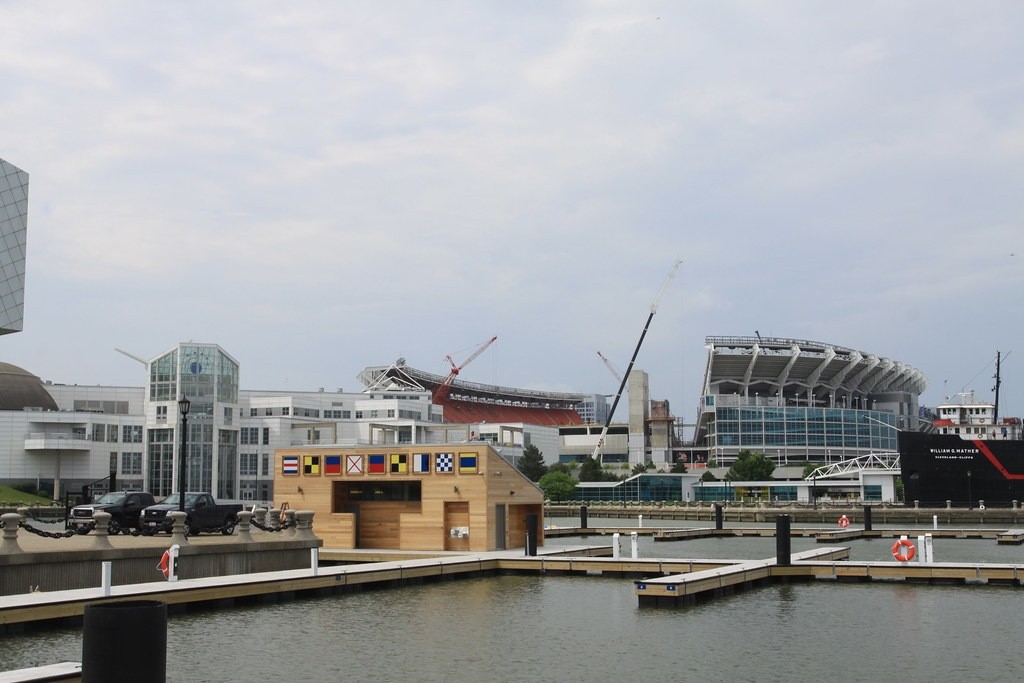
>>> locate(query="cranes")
[597,349,628,391]
[431,335,498,407]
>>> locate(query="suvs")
[69,487,157,539]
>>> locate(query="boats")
[895,428,1024,510]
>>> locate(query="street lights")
[178,390,191,514]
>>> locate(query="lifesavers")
[161,550,169,578]
[891,541,915,562]
[838,518,849,528]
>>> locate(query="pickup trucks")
[139,491,247,537]
